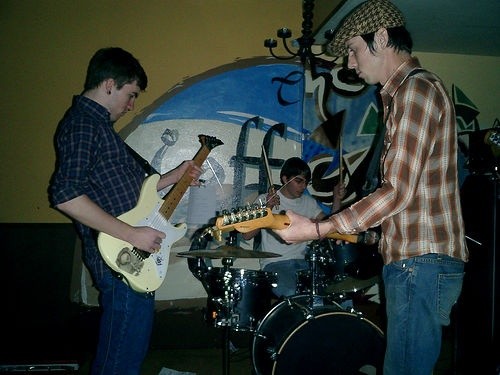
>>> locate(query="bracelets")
[314,219,321,240]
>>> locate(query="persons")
[231,157,347,337]
[270,0,468,375]
[51,47,205,375]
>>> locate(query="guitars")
[484,130,500,153]
[96,134,225,294]
[216,201,385,246]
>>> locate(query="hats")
[327,0,405,56]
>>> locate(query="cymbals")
[175,244,284,258]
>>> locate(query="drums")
[202,265,279,331]
[252,293,388,375]
[302,207,382,295]
[295,268,367,299]
